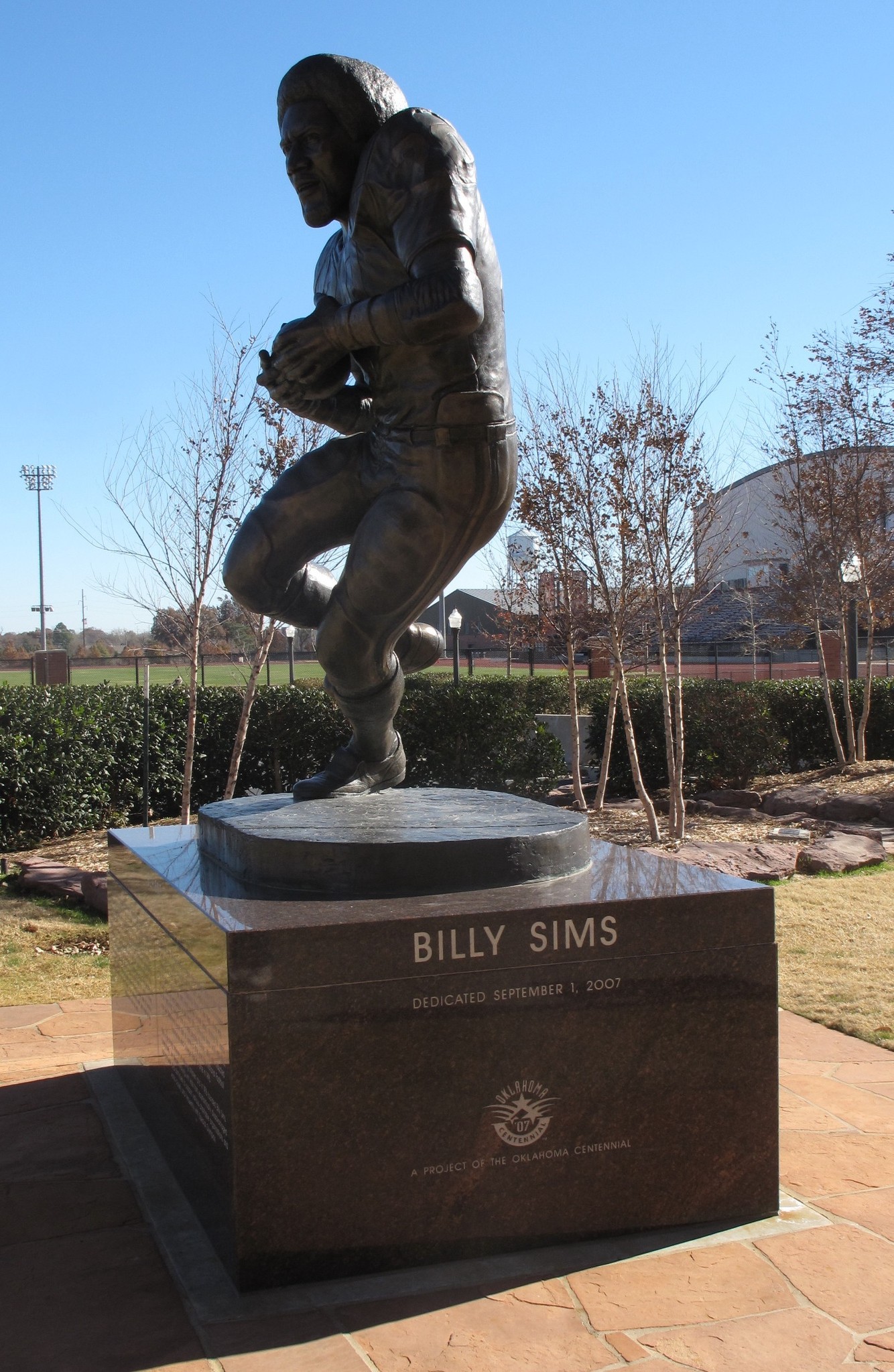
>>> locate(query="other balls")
[269,318,352,402]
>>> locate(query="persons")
[221,56,521,803]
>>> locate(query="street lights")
[285,624,299,684]
[20,462,57,652]
[842,548,866,683]
[448,605,463,684]
